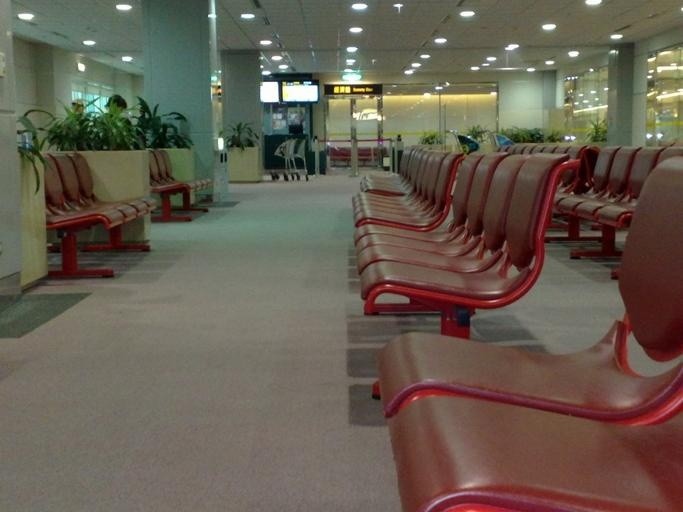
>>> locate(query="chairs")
[38,152,159,280]
[145,148,209,224]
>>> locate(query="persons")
[106,95,132,127]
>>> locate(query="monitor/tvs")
[281,79,319,103]
[260,81,280,104]
